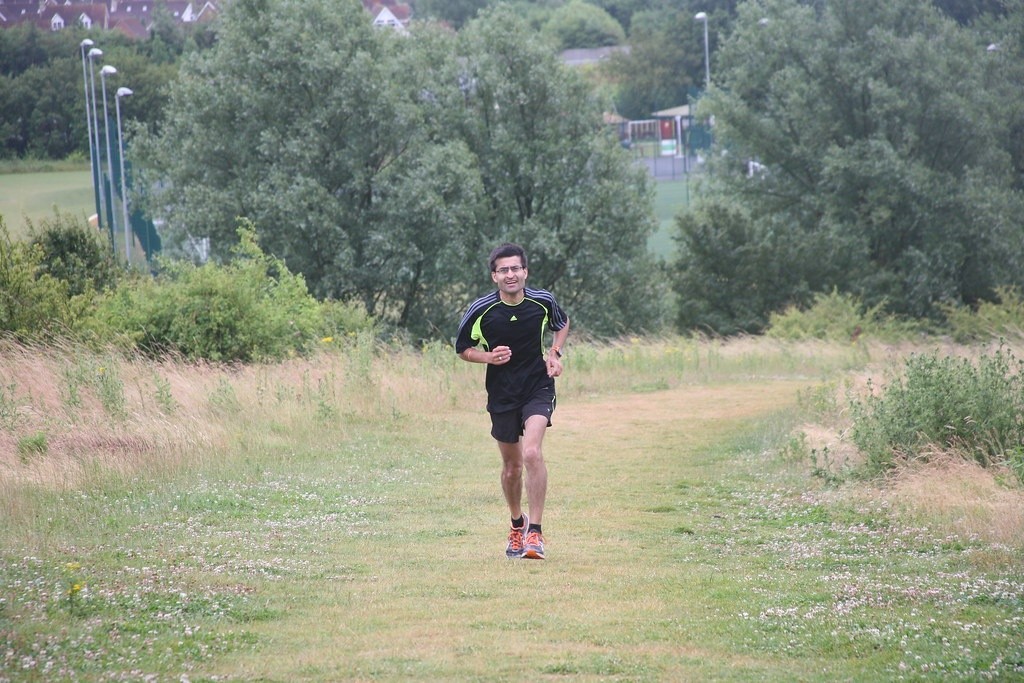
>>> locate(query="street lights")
[78,38,134,271]
[695,10,711,94]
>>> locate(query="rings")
[499,357,501,360]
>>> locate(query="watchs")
[552,346,563,357]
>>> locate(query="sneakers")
[520,528,545,559]
[505,512,529,559]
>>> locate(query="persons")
[455,243,570,559]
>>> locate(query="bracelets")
[468,349,474,360]
